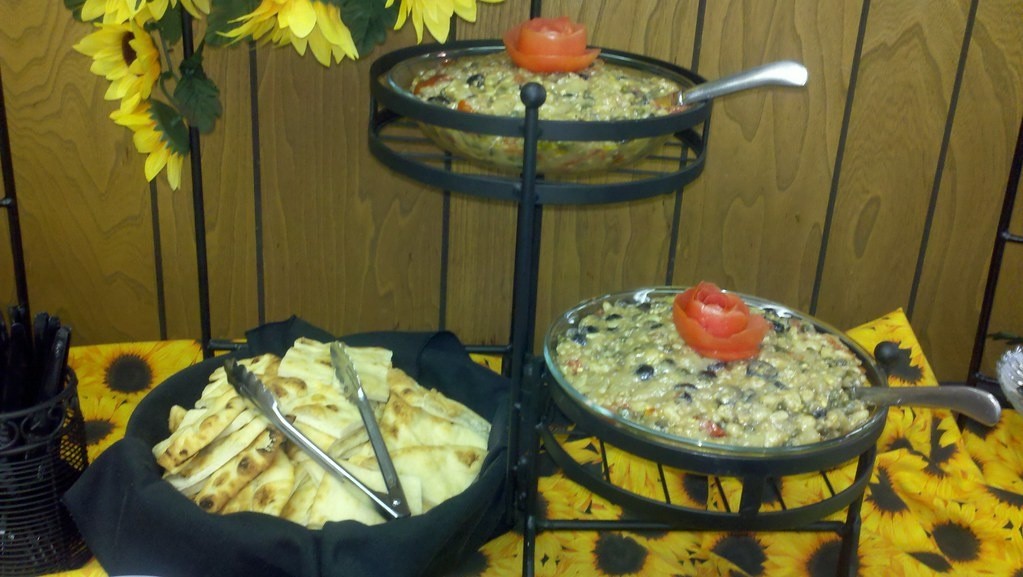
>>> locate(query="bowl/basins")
[542,284,890,459]
[386,47,700,178]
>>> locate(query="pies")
[152,337,492,530]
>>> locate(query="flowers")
[63,0,515,194]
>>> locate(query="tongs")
[222,338,413,521]
